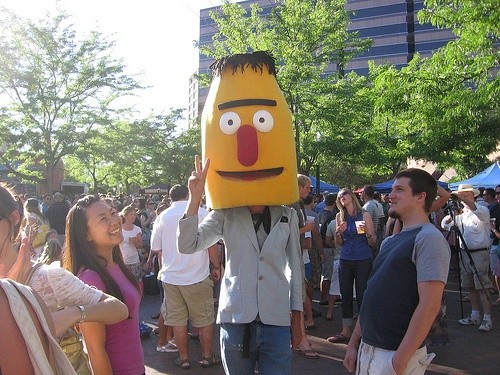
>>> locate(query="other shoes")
[492,297,500,311]
[189,332,199,341]
[457,295,471,302]
[156,340,179,352]
[312,309,323,317]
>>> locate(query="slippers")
[295,348,320,359]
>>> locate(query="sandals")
[327,332,351,343]
[304,320,317,330]
[176,358,191,368]
[201,352,222,368]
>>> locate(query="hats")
[452,183,480,198]
[152,194,159,201]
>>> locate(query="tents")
[449,162,500,190]
[373,178,447,193]
[309,175,339,193]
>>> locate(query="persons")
[343,167,451,375]
[393,185,450,235]
[0,278,55,375]
[178,50,305,375]
[149,186,221,368]
[0,184,129,375]
[101,192,178,351]
[432,184,500,331]
[17,192,88,268]
[65,194,146,375]
[190,198,225,340]
[287,175,402,358]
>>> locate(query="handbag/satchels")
[142,271,161,295]
[59,334,94,375]
[30,212,51,248]
[142,228,150,241]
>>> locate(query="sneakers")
[458,314,482,325]
[478,319,494,332]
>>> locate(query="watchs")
[76,305,87,323]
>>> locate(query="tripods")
[442,202,492,318]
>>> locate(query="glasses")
[337,191,349,199]
[150,203,155,205]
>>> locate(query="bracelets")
[366,234,372,238]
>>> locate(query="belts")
[242,316,260,358]
[459,248,488,252]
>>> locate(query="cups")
[355,221,366,234]
[307,216,316,222]
